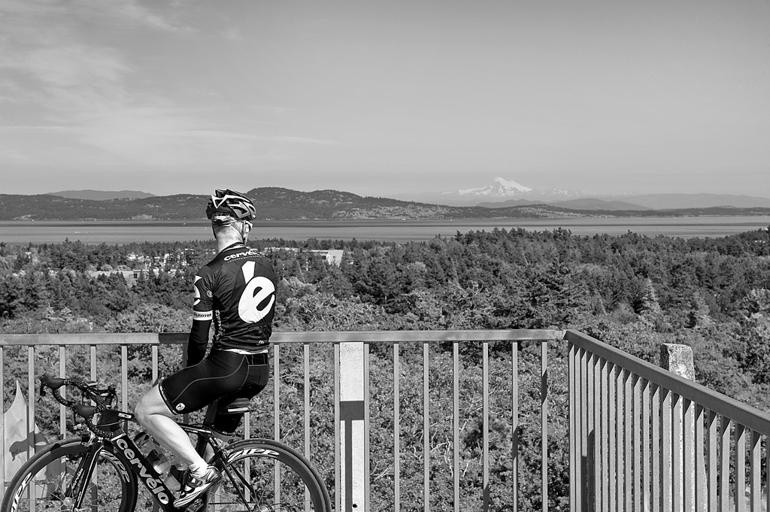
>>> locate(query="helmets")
[206,188,256,221]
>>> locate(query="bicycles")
[1,373,333,512]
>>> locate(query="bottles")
[134,429,172,476]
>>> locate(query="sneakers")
[174,466,222,508]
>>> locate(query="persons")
[133,188,277,507]
